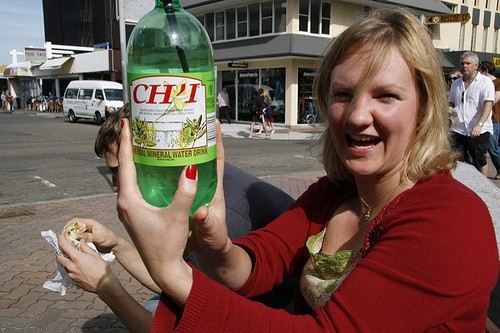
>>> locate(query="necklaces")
[356,179,405,222]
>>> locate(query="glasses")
[108,167,119,177]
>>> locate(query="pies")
[64,221,86,240]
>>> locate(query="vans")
[224,84,285,117]
[62,80,124,126]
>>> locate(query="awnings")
[39,57,71,69]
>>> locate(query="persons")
[53,105,206,333]
[442,51,500,180]
[0,90,7,110]
[249,88,280,140]
[217,87,233,125]
[27,92,64,113]
[115,4,500,333]
[6,92,14,112]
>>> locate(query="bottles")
[126,0,218,218]
